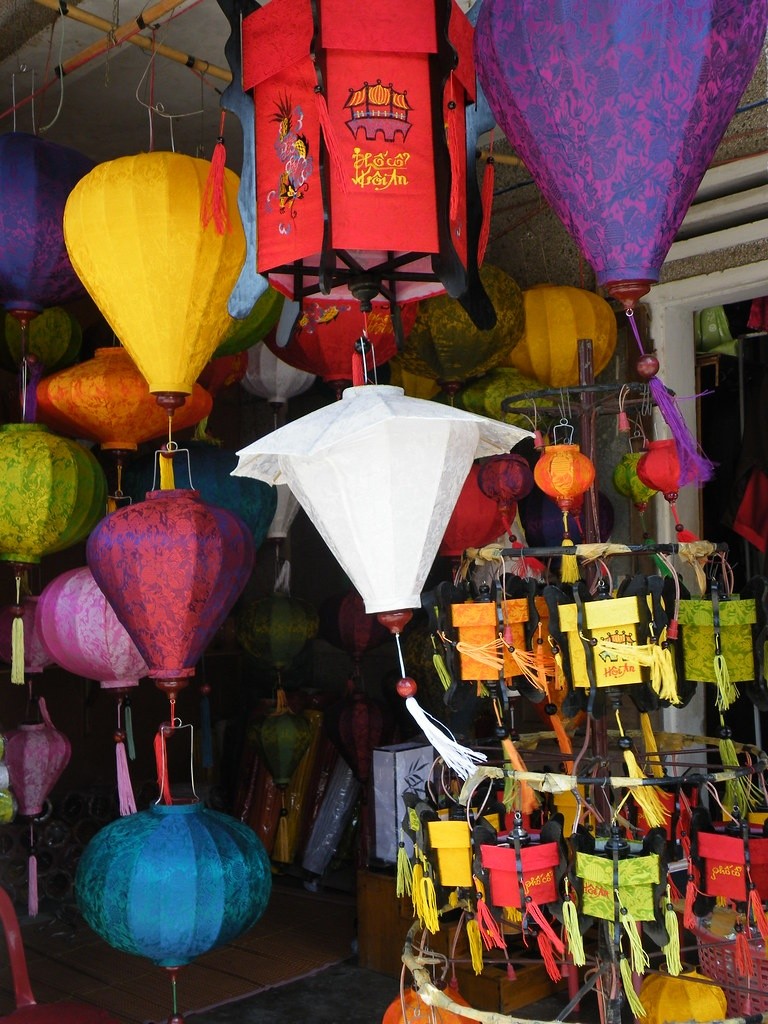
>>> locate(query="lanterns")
[471,1,768,488]
[0,131,768,1024]
[214,1,499,348]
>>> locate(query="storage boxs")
[684,916,768,1020]
